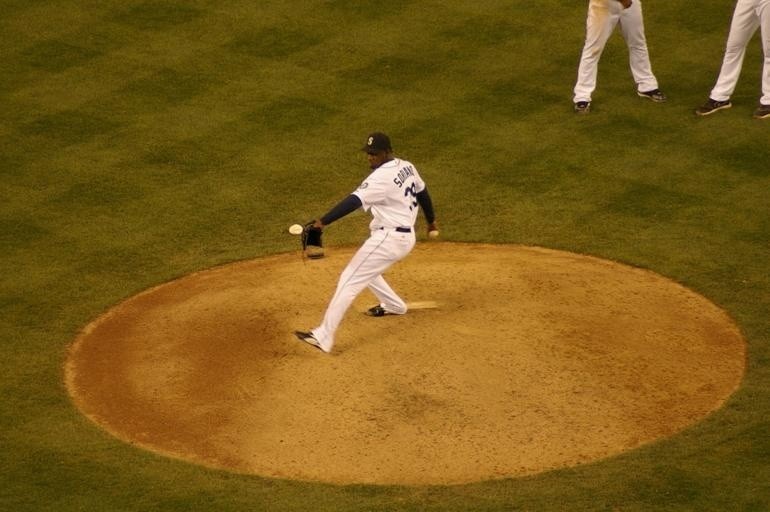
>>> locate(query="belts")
[379,226,412,233]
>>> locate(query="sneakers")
[752,104,770,119]
[573,100,591,116]
[364,304,404,317]
[694,97,733,116]
[636,89,668,104]
[294,329,329,355]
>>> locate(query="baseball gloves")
[300,220,323,250]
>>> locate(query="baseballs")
[429,228,440,239]
[290,223,302,236]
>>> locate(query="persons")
[295,132,439,354]
[695,0,770,120]
[573,0,667,115]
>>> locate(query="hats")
[361,132,392,155]
